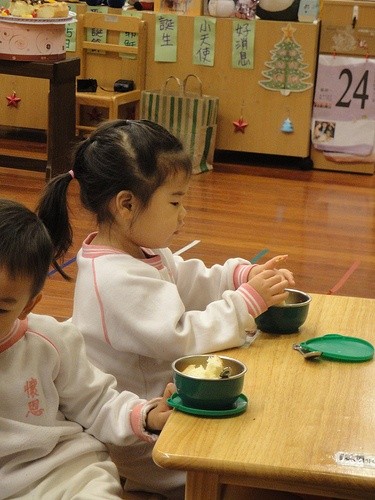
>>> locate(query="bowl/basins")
[251,282,311,336]
[172,347,248,410]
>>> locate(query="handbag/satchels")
[138,73,219,175]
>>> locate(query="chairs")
[76,12,147,137]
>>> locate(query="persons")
[34,119,295,500]
[0,199,178,500]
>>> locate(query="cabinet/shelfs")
[311,0,375,175]
[0,3,321,184]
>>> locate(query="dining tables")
[152,293,375,500]
